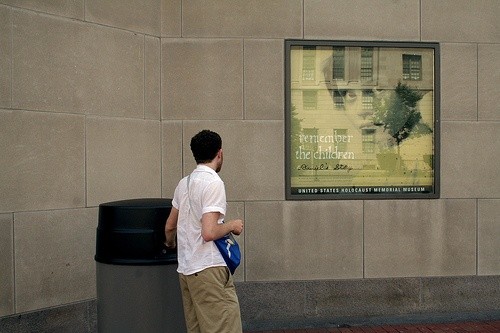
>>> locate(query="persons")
[164,129,243,333]
[322,51,433,186]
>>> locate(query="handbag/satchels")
[214,232,241,275]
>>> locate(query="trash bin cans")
[94,198,187,333]
